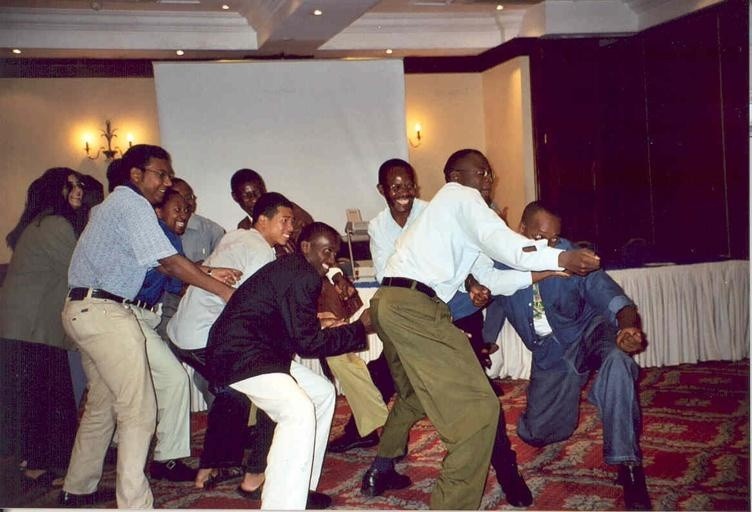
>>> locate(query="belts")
[132,299,156,311]
[382,277,434,297]
[70,288,127,303]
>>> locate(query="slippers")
[194,467,240,490]
[238,478,264,499]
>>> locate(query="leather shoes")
[329,428,380,452]
[498,462,533,506]
[361,470,411,496]
[59,490,115,506]
[306,492,331,509]
[150,460,197,481]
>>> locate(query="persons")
[58,143,244,507]
[326,157,537,509]
[207,222,376,508]
[365,147,602,508]
[481,198,652,508]
[2,167,408,490]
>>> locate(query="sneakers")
[623,472,651,511]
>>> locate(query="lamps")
[82,120,133,163]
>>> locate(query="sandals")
[20,470,63,488]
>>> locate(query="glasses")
[144,168,166,179]
